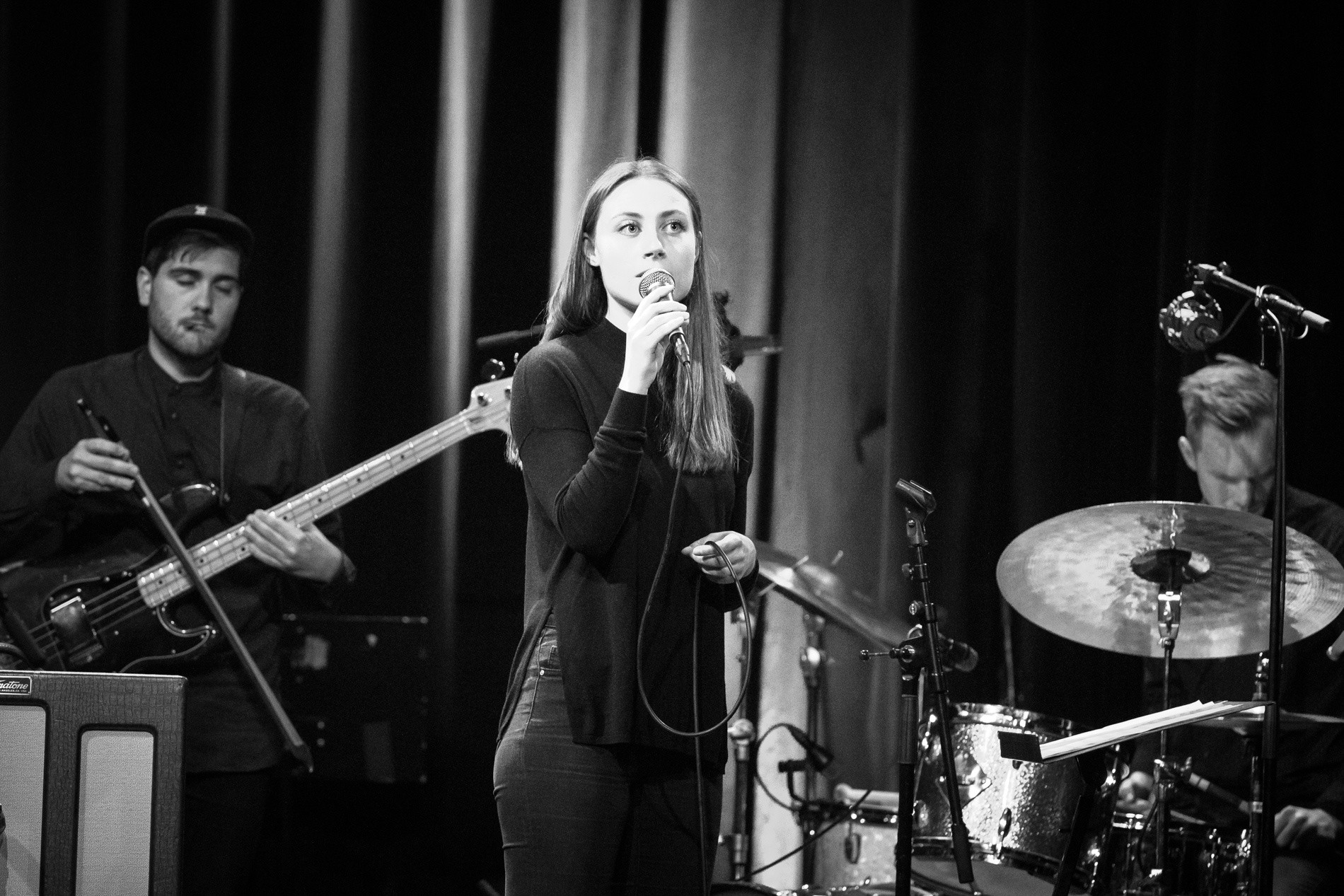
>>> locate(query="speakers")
[0,670,188,896]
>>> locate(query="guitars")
[2,357,515,677]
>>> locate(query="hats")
[141,203,255,269]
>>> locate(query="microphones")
[1159,300,1222,351]
[638,268,690,365]
[786,725,834,772]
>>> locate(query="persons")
[1099,355,1344,856]
[493,158,754,896]
[0,203,355,896]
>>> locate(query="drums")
[908,700,1080,896]
[1071,810,1225,896]
[807,799,899,896]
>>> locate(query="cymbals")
[994,500,1344,660]
[749,539,918,655]
[1188,703,1343,731]
